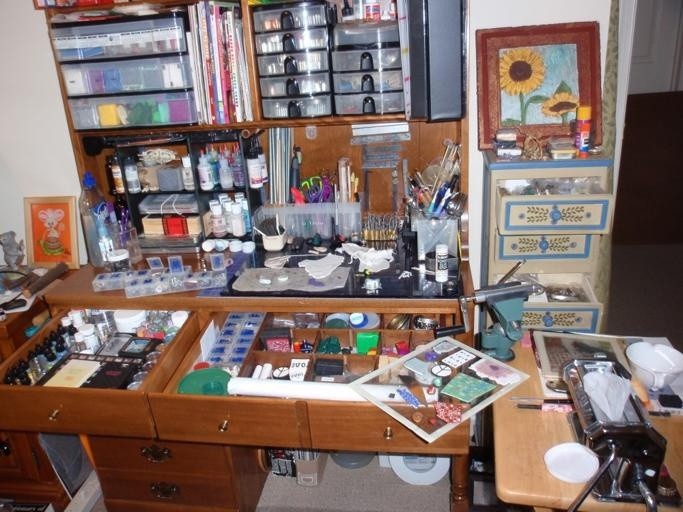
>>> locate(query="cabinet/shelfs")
[45,0,468,251]
[480,152,614,350]
[0,273,92,512]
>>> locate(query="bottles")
[254,137,268,183]
[246,141,263,190]
[105,248,130,272]
[341,1,354,21]
[113,194,128,220]
[388,3,396,20]
[181,156,194,191]
[195,144,243,190]
[353,0,366,20]
[105,150,141,196]
[3,311,100,385]
[209,193,252,237]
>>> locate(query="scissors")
[290,176,335,203]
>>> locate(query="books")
[186,0,255,125]
[265,126,295,206]
[531,330,630,381]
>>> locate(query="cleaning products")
[95,212,115,270]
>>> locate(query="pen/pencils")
[117,208,128,245]
[403,143,462,219]
[516,403,542,410]
[545,399,574,403]
[510,396,545,401]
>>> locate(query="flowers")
[497,42,581,135]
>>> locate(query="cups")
[625,341,683,392]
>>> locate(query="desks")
[489,329,683,512]
[0,251,476,511]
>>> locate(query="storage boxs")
[263,190,366,240]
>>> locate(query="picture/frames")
[24,194,80,270]
[474,22,605,153]
